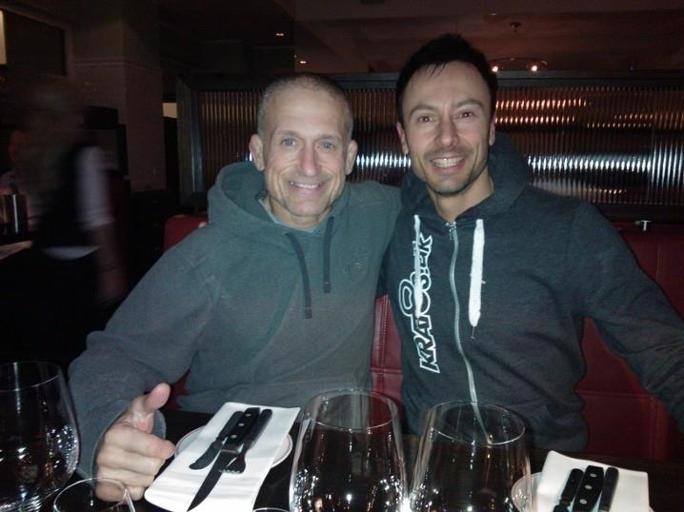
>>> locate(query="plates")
[173,424,293,472]
[511,471,545,512]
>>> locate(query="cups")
[5,191,29,241]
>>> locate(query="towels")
[144,400,302,512]
[529,449,650,512]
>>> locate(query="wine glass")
[54,478,137,512]
[1,359,80,512]
[409,400,532,512]
[288,390,409,512]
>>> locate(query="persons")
[65,73,399,503]
[376,32,684,452]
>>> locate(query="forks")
[218,409,272,474]
[598,467,620,512]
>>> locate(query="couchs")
[163,214,683,460]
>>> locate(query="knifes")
[553,468,583,512]
[189,411,245,470]
[186,406,261,512]
[571,466,603,511]
